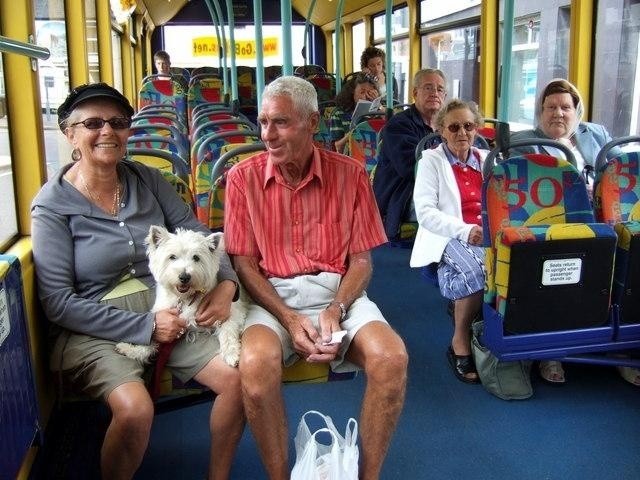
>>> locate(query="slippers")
[446,345,479,384]
[615,354,640,386]
[540,360,566,386]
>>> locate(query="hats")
[58,82,134,135]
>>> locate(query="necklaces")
[77,161,121,215]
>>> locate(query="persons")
[223,75,409,480]
[329,47,639,386]
[29,80,247,480]
[145,51,189,94]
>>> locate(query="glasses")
[70,118,132,129]
[448,122,477,132]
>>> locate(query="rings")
[178,327,186,335]
[214,320,222,328]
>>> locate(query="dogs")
[113,225,248,367]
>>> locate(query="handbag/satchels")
[471,321,534,400]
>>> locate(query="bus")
[39,68,97,115]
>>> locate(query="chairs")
[121,65,360,416]
[473,135,639,369]
[360,65,518,243]
